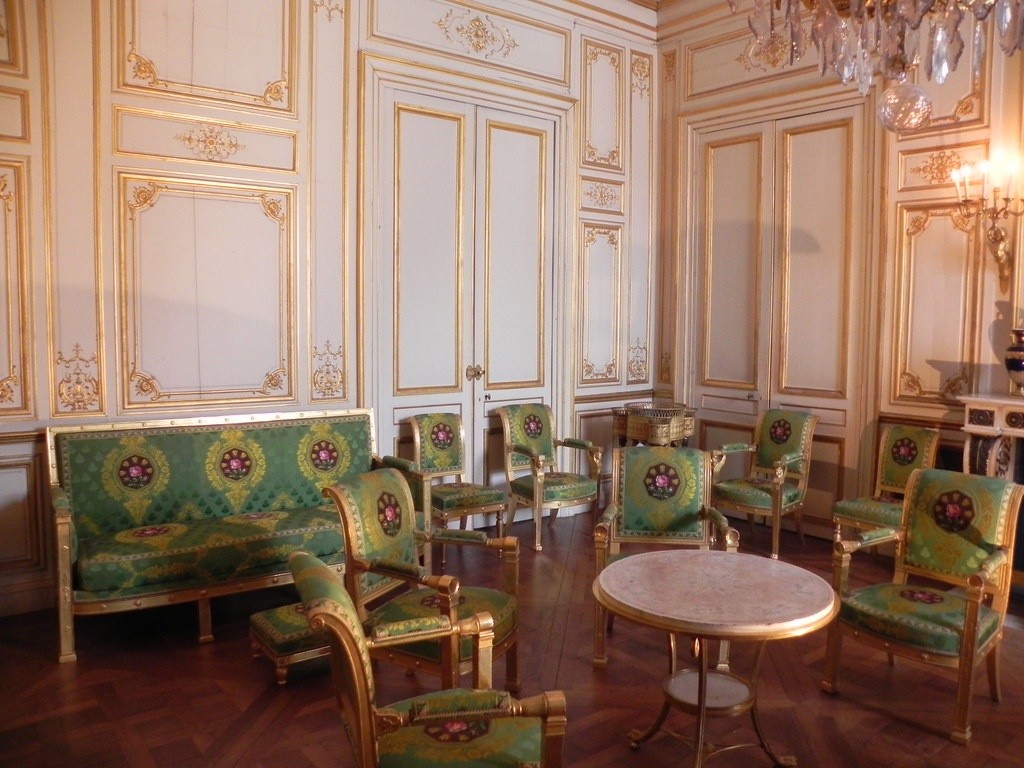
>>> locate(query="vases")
[1005,328,1024,397]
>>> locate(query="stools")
[247,602,335,685]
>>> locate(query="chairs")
[321,466,524,699]
[495,403,604,552]
[711,409,820,560]
[594,446,740,658]
[287,551,568,768]
[831,424,942,551]
[820,468,1024,747]
[408,413,505,571]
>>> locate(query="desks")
[590,548,839,768]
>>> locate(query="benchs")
[42,406,430,665]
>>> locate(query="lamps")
[730,0,1024,137]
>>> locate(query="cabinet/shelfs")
[956,393,1024,480]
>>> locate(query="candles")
[982,172,989,199]
[1006,172,1018,200]
[952,170,963,204]
[964,165,972,198]
